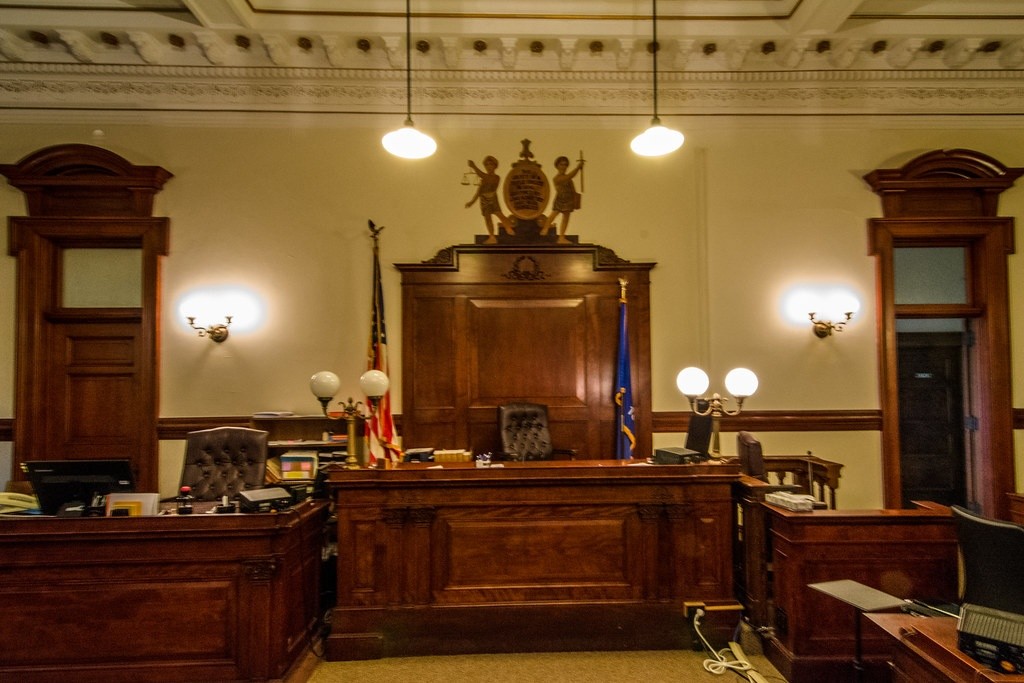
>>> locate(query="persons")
[540,157,582,244]
[464,155,515,245]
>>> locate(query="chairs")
[951,505,1024,616]
[160,426,270,502]
[735,431,772,485]
[498,403,581,460]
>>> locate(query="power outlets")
[687,606,705,619]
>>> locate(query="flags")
[615,298,636,458]
[365,237,403,465]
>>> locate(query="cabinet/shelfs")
[249,415,365,466]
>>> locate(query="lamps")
[309,369,388,472]
[675,367,759,460]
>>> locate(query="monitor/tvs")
[26,457,135,517]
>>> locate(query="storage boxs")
[281,449,318,480]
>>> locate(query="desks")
[758,498,962,683]
[0,500,328,683]
[862,609,1024,683]
[323,457,745,661]
